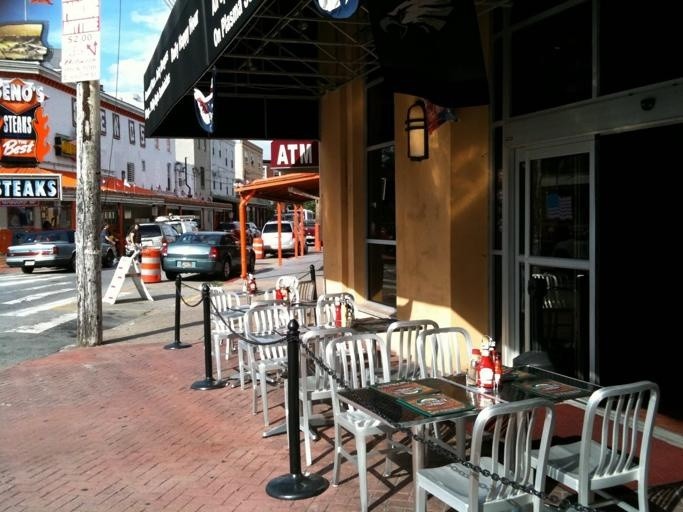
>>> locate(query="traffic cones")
[252,238,264,259]
[140,249,161,283]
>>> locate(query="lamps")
[405,102,429,161]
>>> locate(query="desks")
[335,365,605,512]
[214,300,319,388]
[261,318,422,440]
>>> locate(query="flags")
[29,0,55,6]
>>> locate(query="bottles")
[333,297,353,329]
[242,272,257,296]
[466,349,480,386]
[275,285,291,306]
[477,350,495,389]
[494,355,502,385]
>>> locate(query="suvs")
[302,218,315,243]
[246,221,261,237]
[261,219,307,256]
[217,221,239,232]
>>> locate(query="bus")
[288,209,313,223]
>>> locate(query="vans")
[285,213,299,222]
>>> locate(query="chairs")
[326,334,389,512]
[237,303,268,390]
[277,276,297,302]
[284,329,356,466]
[211,292,241,380]
[528,383,658,511]
[417,399,557,512]
[243,306,291,425]
[220,293,241,350]
[317,294,355,325]
[293,280,315,323]
[365,321,439,384]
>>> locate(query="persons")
[100,223,121,244]
[130,224,142,264]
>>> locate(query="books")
[396,393,474,418]
[509,377,584,400]
[369,378,441,401]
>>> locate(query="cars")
[162,231,256,280]
[164,216,201,240]
[6,230,116,274]
[127,223,179,256]
